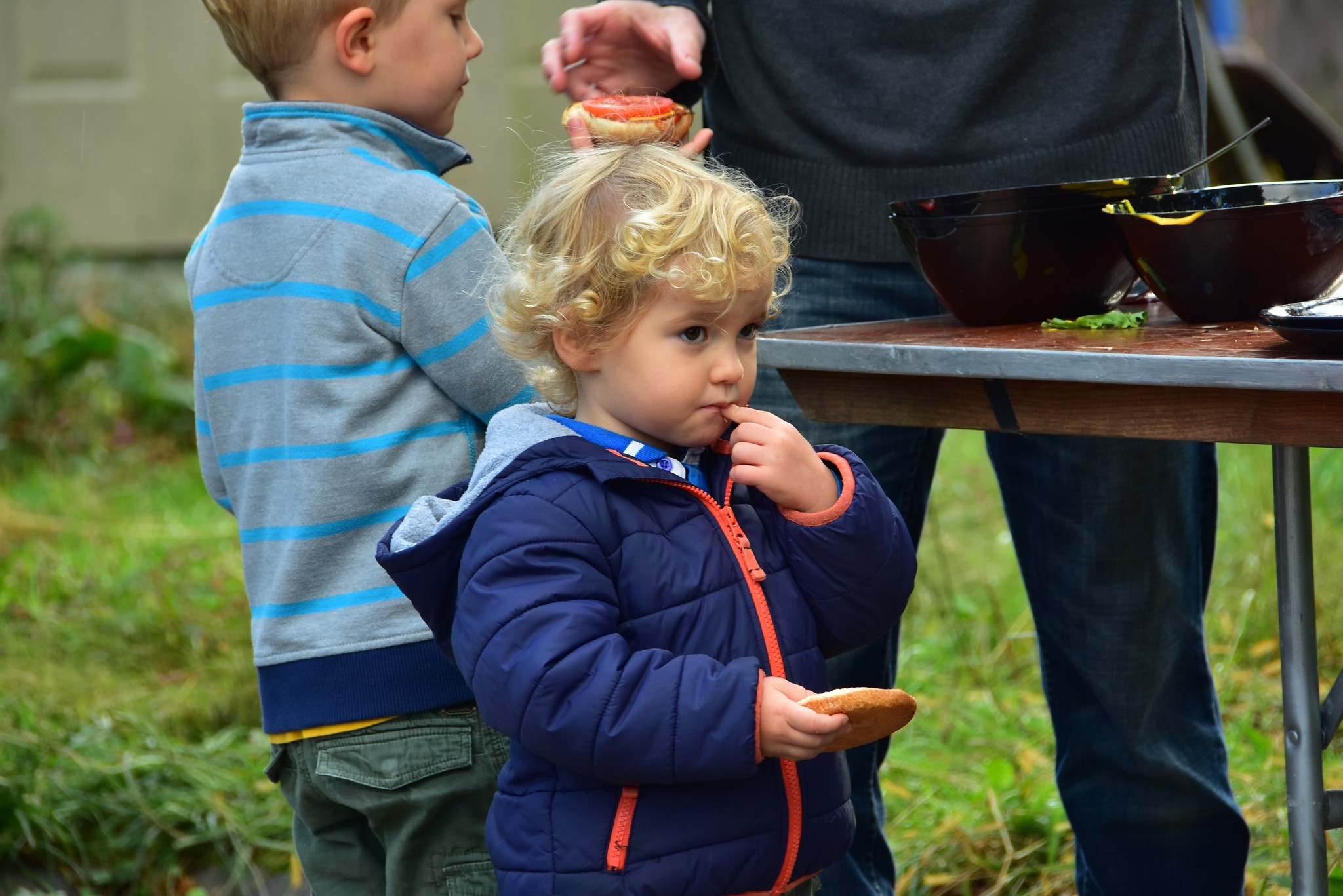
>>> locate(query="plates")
[1260,297,1342,355]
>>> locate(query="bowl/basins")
[887,174,1185,325]
[1100,180,1343,325]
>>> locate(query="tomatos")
[580,95,673,118]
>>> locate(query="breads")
[793,687,917,753]
[561,100,695,147]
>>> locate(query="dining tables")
[758,289,1343,896]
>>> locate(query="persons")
[181,0,548,896]
[537,0,1251,896]
[374,140,918,896]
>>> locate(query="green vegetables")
[1040,309,1147,330]
